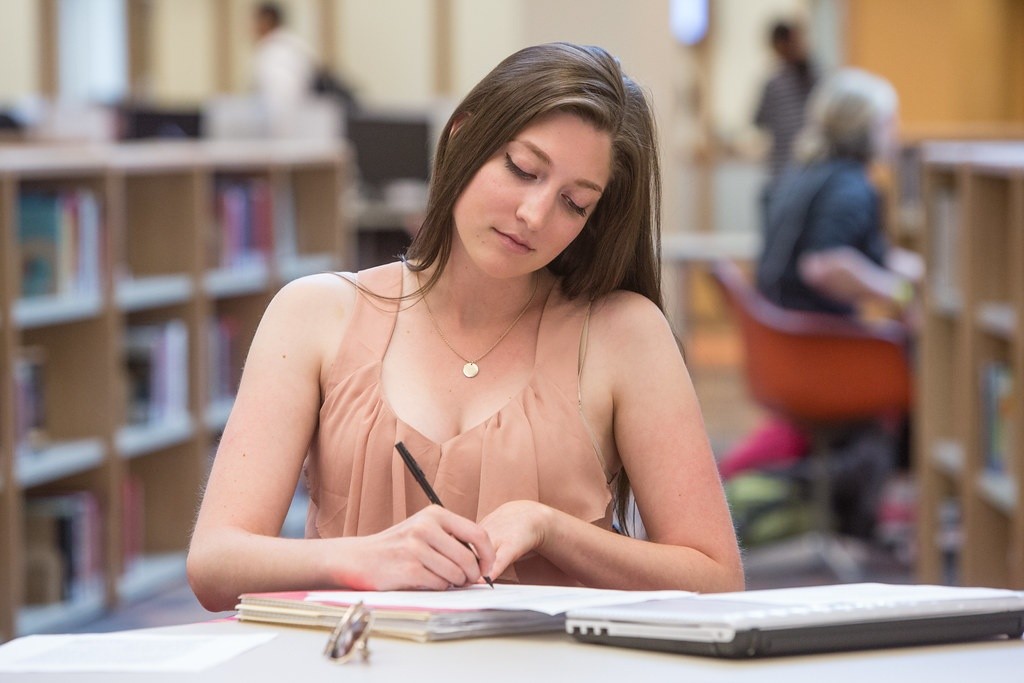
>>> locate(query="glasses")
[324,600,375,663]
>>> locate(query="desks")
[1,619,1024,682]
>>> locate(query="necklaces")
[415,259,537,375]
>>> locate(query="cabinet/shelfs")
[898,135,1023,594]
[0,137,354,640]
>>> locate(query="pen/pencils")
[395,442,495,590]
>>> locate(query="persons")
[189,40,741,592]
[226,0,926,571]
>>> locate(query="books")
[18,174,1013,639]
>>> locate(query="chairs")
[708,259,912,553]
